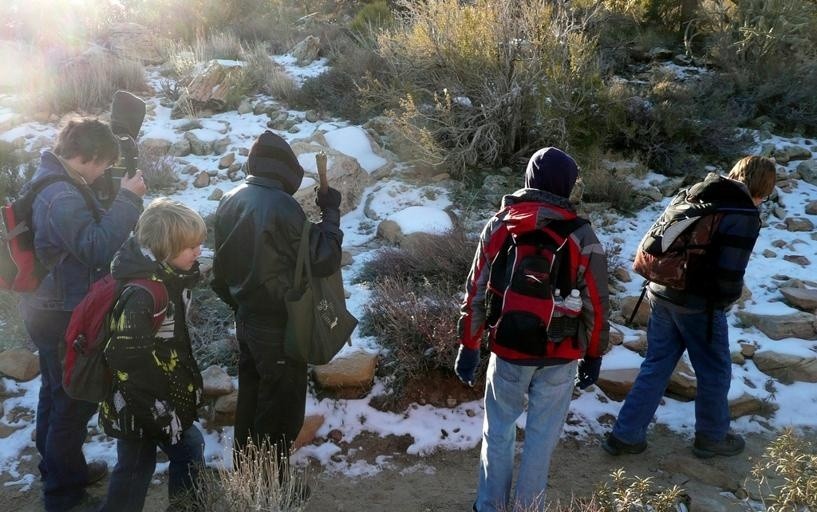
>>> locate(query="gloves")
[576,356,601,391]
[452,344,480,386]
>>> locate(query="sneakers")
[82,460,108,482]
[694,436,745,459]
[607,436,651,452]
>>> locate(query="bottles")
[548,288,567,342]
[564,289,582,338]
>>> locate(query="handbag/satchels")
[283,216,359,364]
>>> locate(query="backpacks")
[61,273,169,402]
[0,173,101,291]
[486,218,591,366]
[632,187,725,292]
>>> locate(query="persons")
[455,147,611,512]
[602,155,775,459]
[97,197,205,512]
[213,130,342,500]
[102,90,146,198]
[22,117,146,512]
